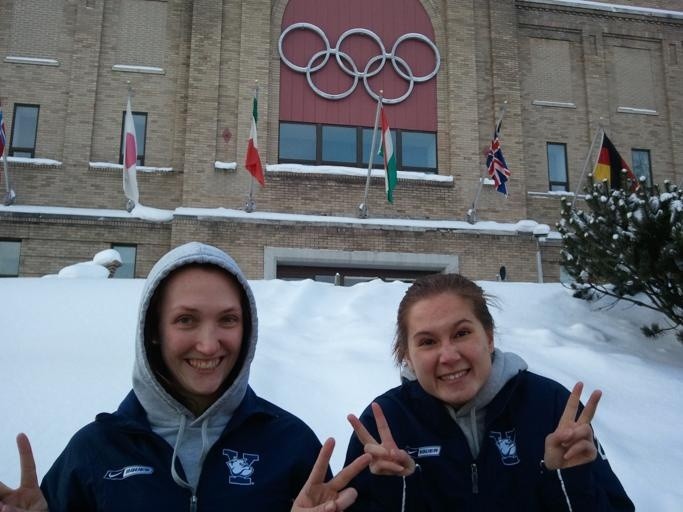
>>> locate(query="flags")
[376,102,397,206]
[589,131,638,192]
[485,118,511,201]
[121,93,140,207]
[0,96,7,159]
[242,86,264,190]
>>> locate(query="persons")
[1,241,376,510]
[338,271,635,511]
